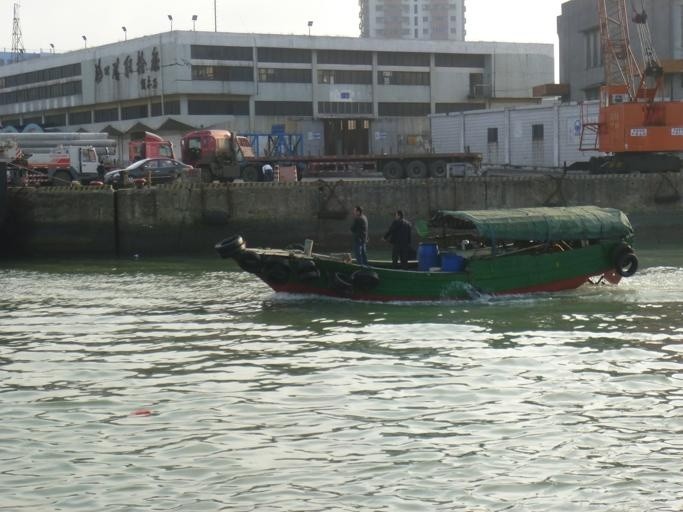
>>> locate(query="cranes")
[577,0,682,154]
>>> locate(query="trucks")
[1,130,176,179]
[180,129,483,182]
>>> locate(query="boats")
[213,203,639,302]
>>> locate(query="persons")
[349,205,370,267]
[380,209,413,270]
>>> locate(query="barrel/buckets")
[441,251,466,272]
[417,241,438,271]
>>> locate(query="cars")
[105,157,193,188]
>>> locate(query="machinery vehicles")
[0,139,104,185]
